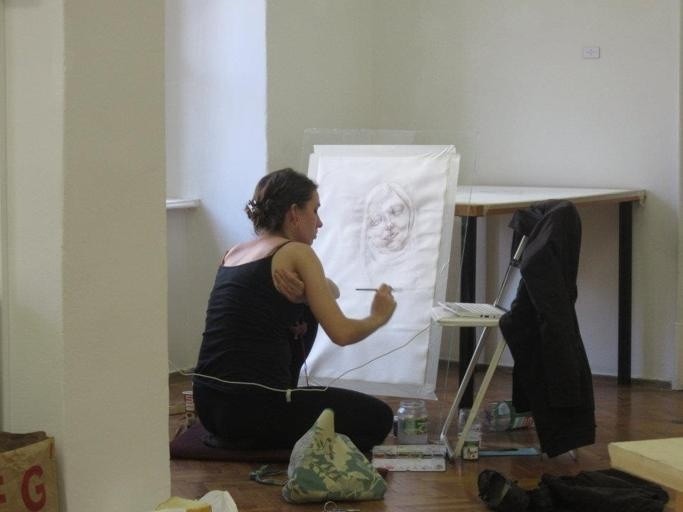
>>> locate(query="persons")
[192,170,397,452]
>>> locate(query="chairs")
[431,200,596,461]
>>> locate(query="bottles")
[481,400,538,430]
[459,407,482,462]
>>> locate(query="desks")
[455,185,646,419]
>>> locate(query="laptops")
[437,259,523,318]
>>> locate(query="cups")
[182,390,198,415]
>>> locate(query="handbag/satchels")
[281,408,388,503]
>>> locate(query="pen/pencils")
[356,287,398,292]
